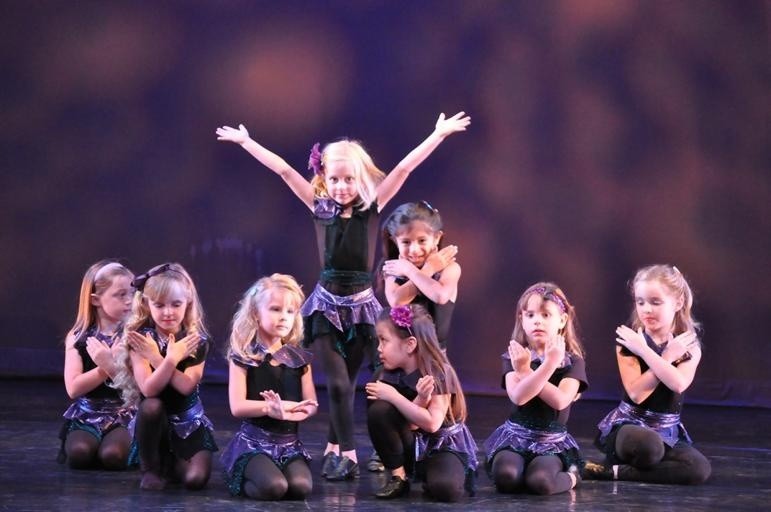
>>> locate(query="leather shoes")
[372,474,414,500]
[580,462,615,482]
[319,448,342,479]
[326,455,362,483]
[139,471,169,492]
[464,469,478,499]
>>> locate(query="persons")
[215,111,471,481]
[219,273,320,498]
[55,261,138,471]
[482,283,590,496]
[367,304,480,500]
[582,264,713,482]
[376,200,462,363]
[110,264,218,491]
